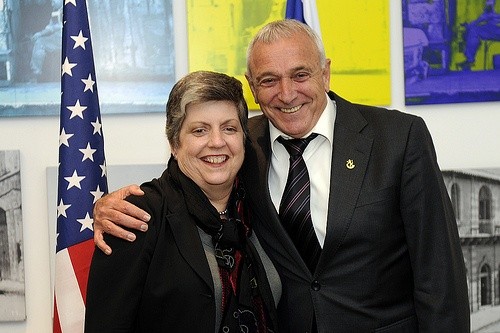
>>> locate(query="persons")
[84,71,283,333]
[93,17,470,333]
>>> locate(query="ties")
[276,133,323,275]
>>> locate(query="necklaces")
[219,208,227,215]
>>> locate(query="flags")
[52,0,107,332]
